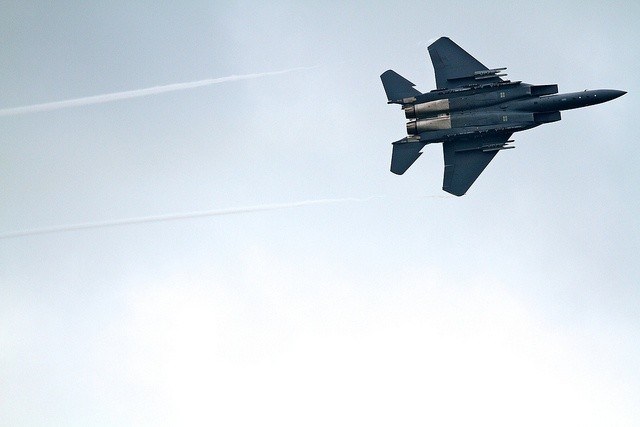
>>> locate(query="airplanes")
[380,36,627,196]
